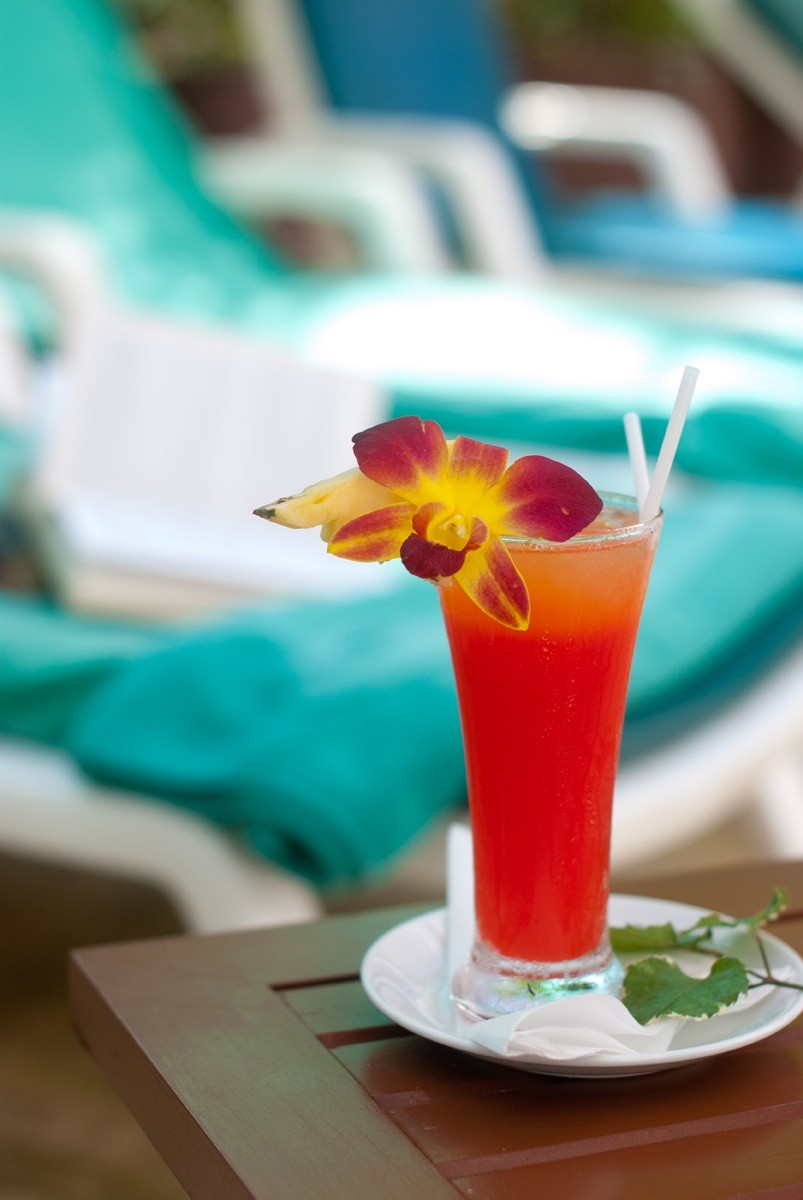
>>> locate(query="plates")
[361,891,802,1082]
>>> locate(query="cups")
[437,494,662,1023]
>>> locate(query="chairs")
[0,2,803,964]
[236,2,803,338]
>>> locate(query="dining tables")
[60,860,803,1200]
